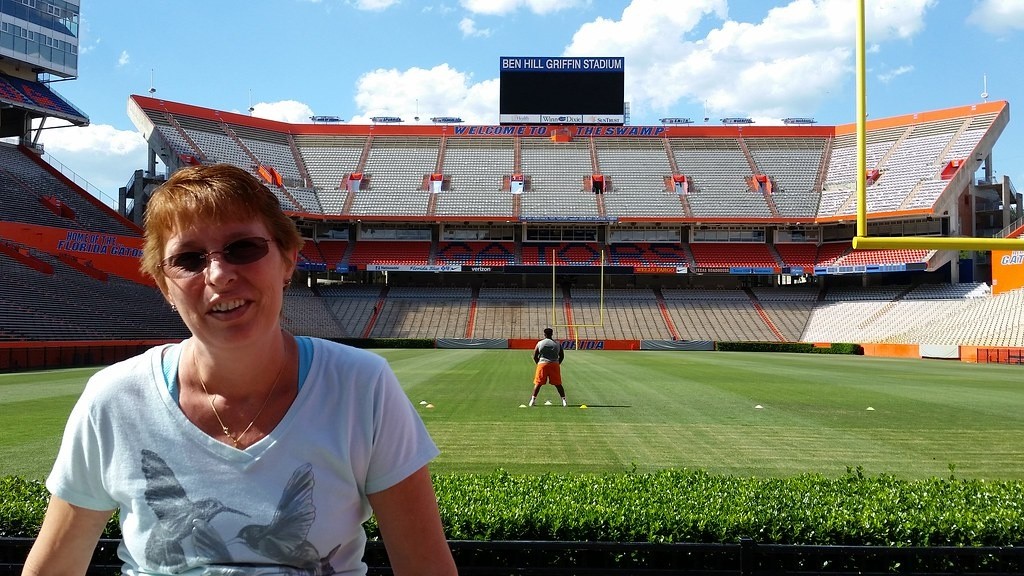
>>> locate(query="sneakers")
[562,401,567,406]
[529,400,535,406]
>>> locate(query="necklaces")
[193,330,287,447]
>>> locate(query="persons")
[21,162,459,576]
[529,328,567,407]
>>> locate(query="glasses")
[157,236,274,279]
